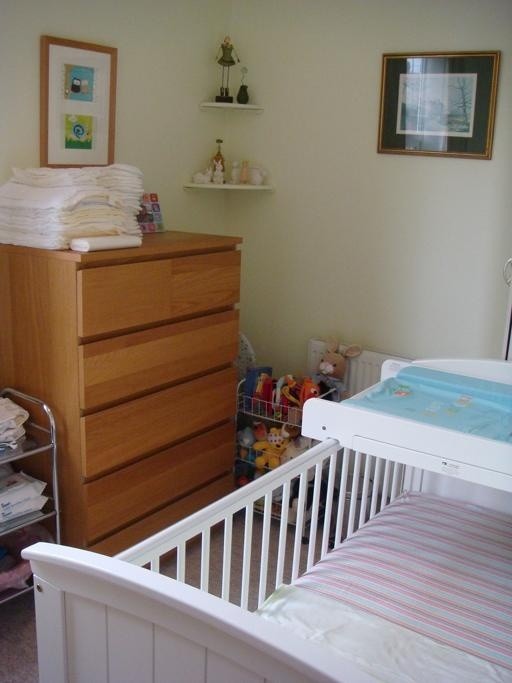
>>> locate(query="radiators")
[304,338,414,500]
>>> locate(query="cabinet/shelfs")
[1,387,62,608]
[1,230,243,569]
[236,376,339,545]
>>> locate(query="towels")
[1,164,146,252]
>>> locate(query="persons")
[213,32,241,103]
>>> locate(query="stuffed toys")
[233,334,364,525]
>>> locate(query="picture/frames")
[37,32,118,168]
[376,50,502,161]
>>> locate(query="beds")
[23,355,511,682]
